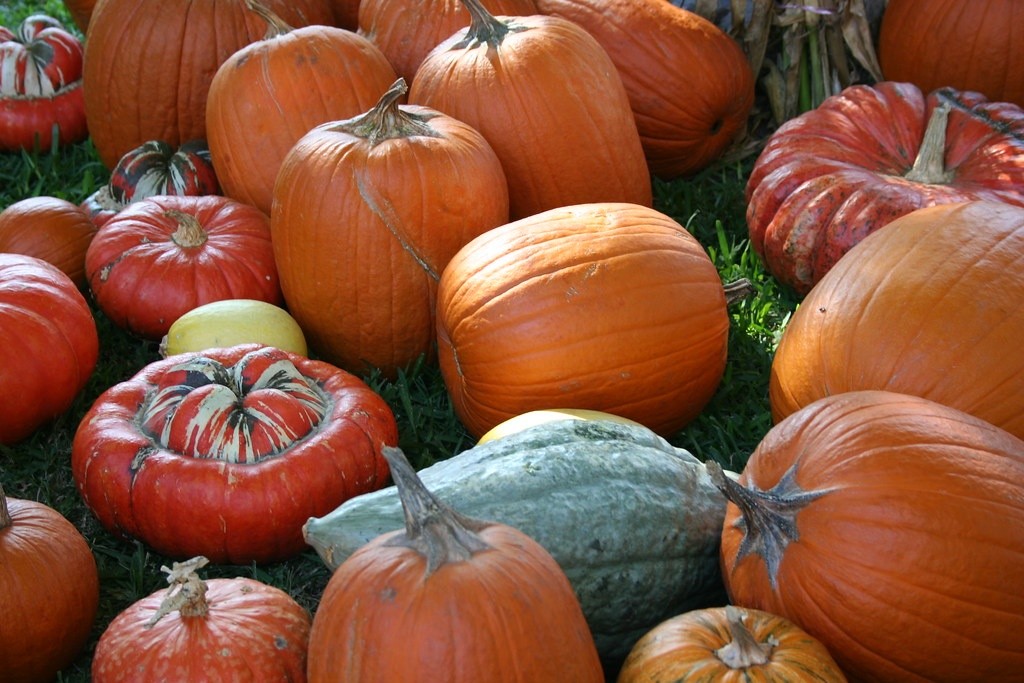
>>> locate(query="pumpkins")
[0,0,1024,683]
